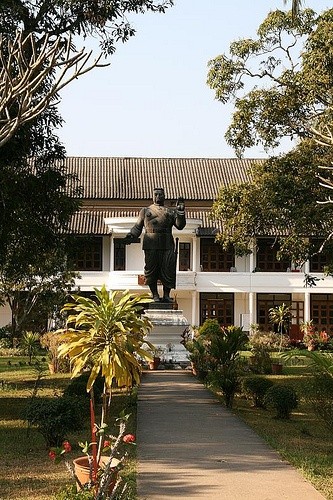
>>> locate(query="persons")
[122,187,186,303]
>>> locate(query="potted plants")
[268,303,295,374]
[49,283,159,495]
[40,326,65,376]
[145,347,163,371]
[302,335,319,351]
